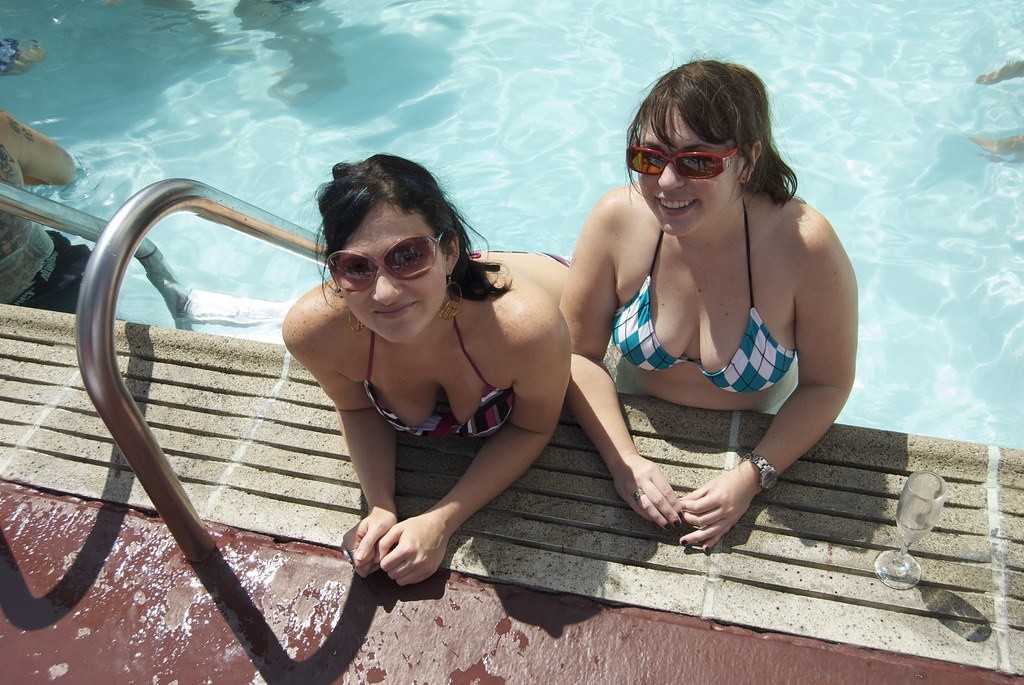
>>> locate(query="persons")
[0,109,76,305]
[559,60,858,556]
[281,153,571,587]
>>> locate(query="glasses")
[327,232,444,292]
[626,139,741,179]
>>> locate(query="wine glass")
[873,471,947,589]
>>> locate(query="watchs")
[742,452,778,489]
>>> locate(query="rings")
[632,489,644,500]
[343,546,358,564]
